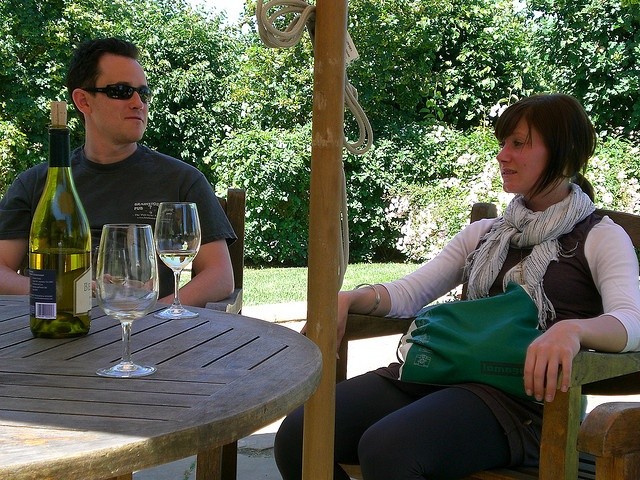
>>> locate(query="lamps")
[106,187,247,480]
[335,201,640,480]
[575,400,640,480]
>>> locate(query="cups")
[81,84,151,103]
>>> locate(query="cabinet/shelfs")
[29,126,93,339]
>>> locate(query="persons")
[0,38,238,308]
[274,95,640,479]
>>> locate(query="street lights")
[396,280,544,401]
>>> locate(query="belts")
[153,202,201,321]
[96,224,159,379]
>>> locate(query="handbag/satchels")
[353,283,381,315]
